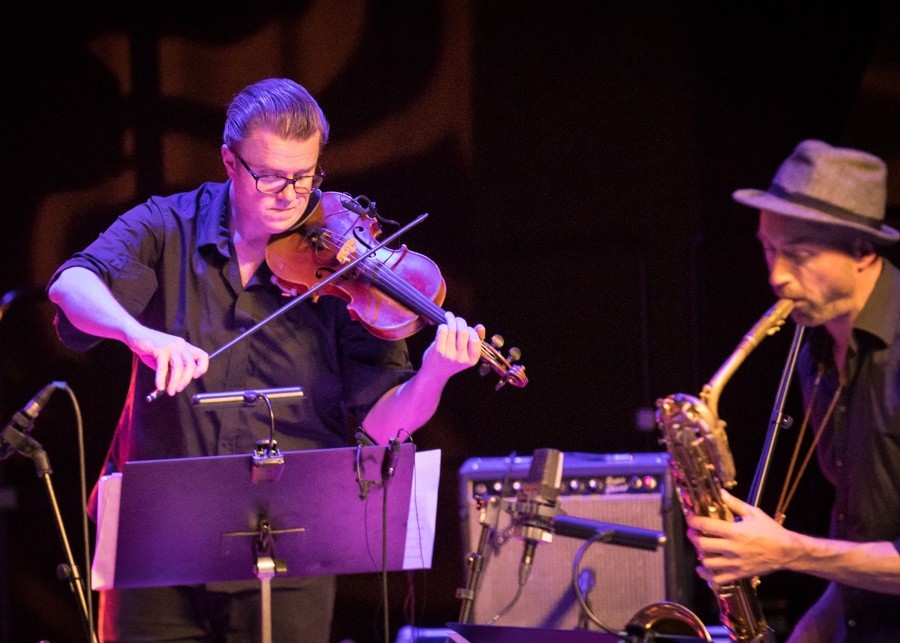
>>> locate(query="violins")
[264,188,529,391]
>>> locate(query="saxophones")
[624,297,796,643]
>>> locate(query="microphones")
[0,381,57,462]
[518,448,564,585]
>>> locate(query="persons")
[687,140,900,643]
[46,80,485,643]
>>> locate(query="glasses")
[230,148,325,194]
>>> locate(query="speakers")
[460,452,698,634]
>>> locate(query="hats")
[734,140,900,240]
